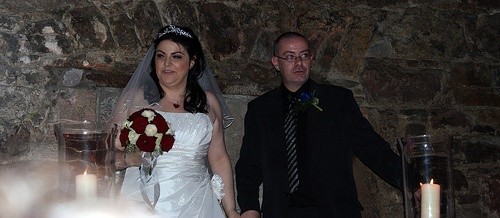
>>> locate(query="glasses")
[275,54,312,62]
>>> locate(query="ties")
[284,96,300,194]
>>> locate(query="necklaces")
[164,96,182,108]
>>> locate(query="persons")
[104,25,240,218]
[235,32,429,218]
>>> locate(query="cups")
[54,122,115,202]
[402,134,456,218]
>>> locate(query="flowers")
[115,108,176,184]
[301,90,324,112]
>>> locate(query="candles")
[421,179,440,218]
[76,172,98,202]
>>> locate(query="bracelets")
[123,151,128,166]
